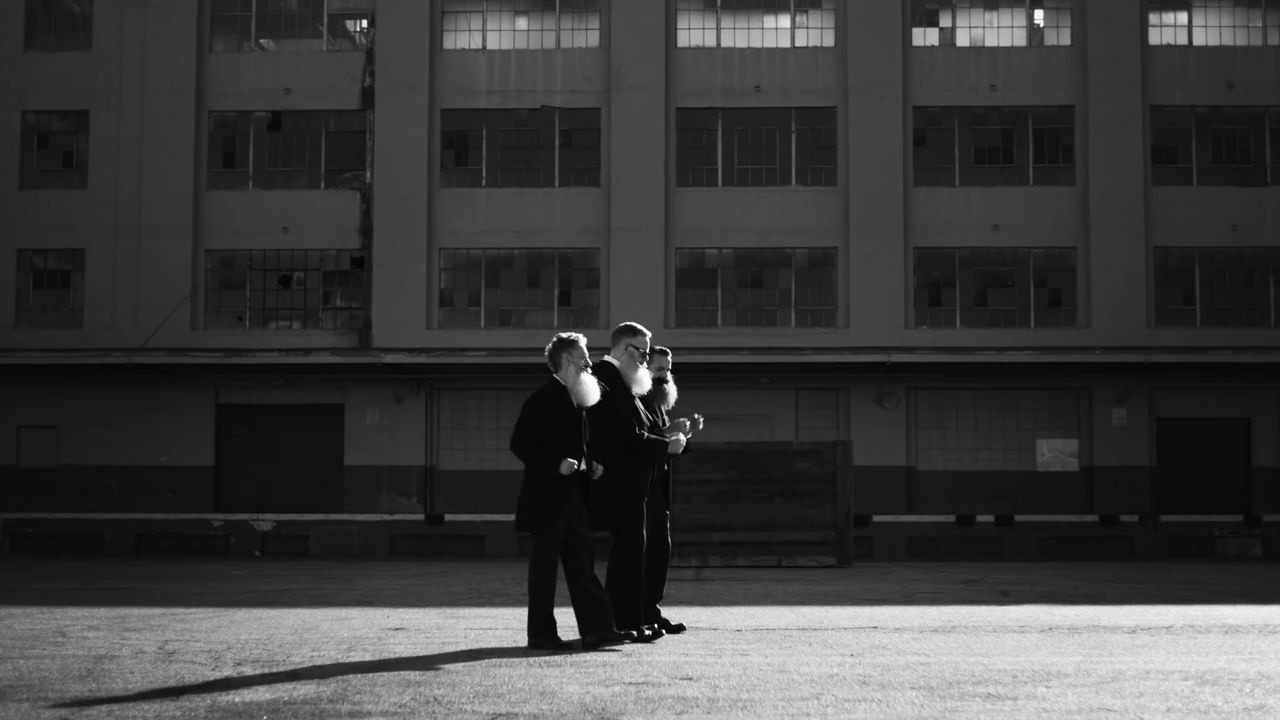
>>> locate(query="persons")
[511,331,634,652]
[588,322,688,644]
[647,346,702,634]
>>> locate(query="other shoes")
[526,635,580,651]
[659,617,688,635]
[584,626,640,647]
[630,628,667,643]
[643,622,659,631]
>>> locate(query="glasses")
[632,344,651,357]
